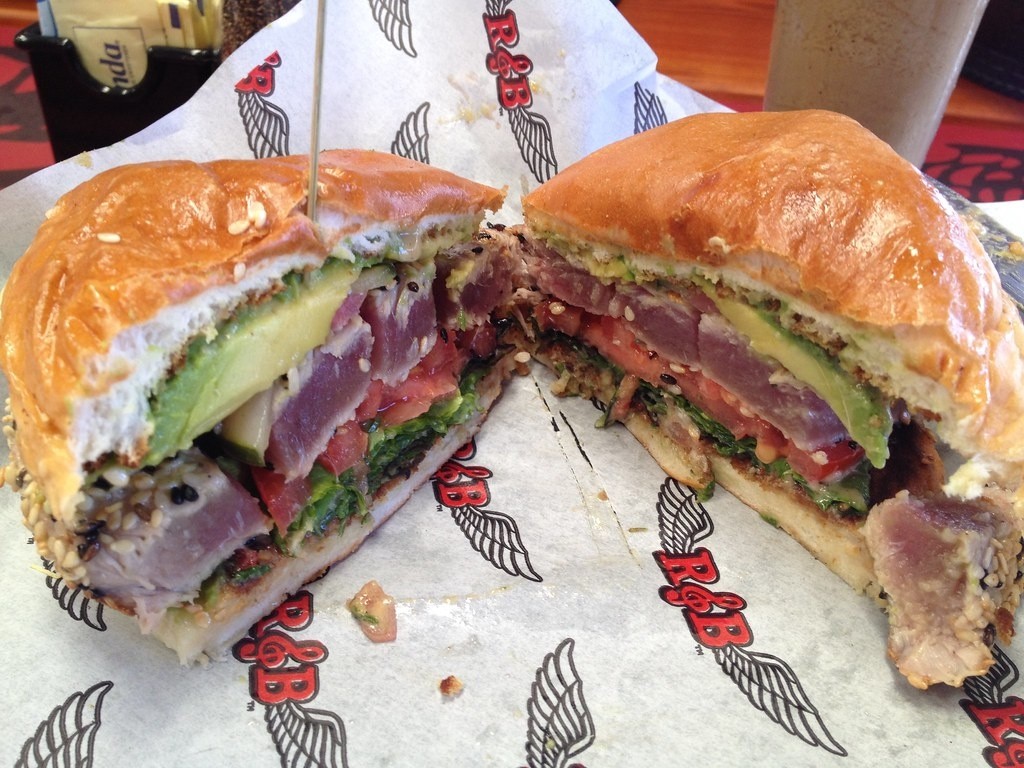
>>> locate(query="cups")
[763,0,990,172]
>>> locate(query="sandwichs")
[3,108,1024,688]
